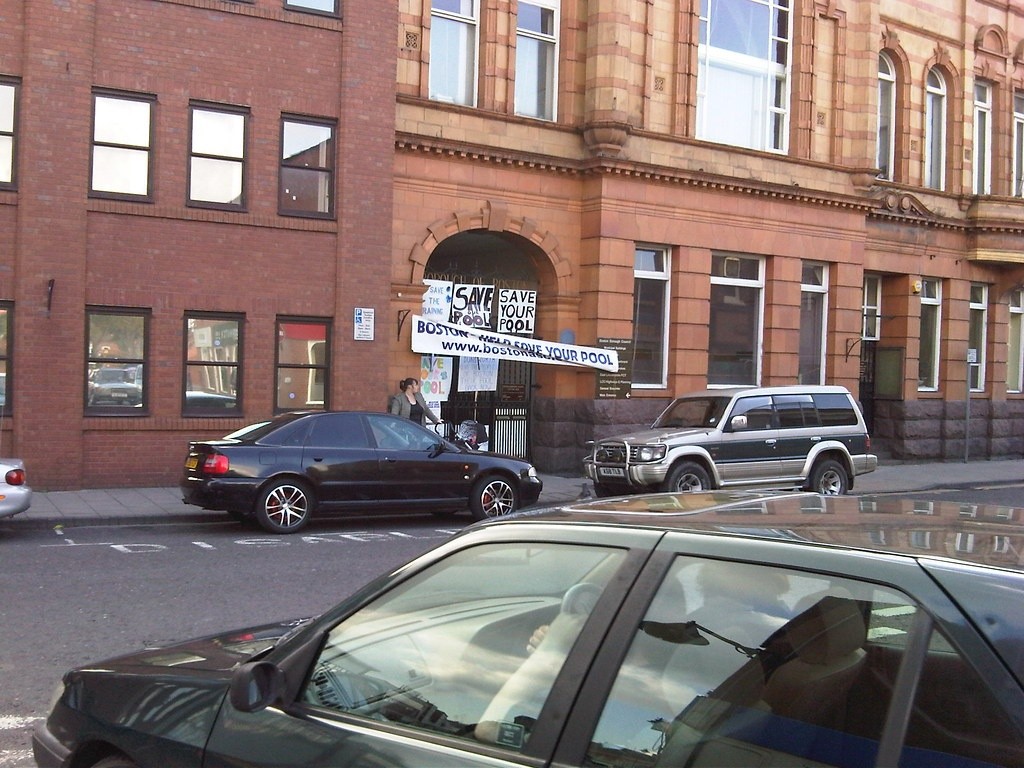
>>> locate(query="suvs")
[583,385,876,500]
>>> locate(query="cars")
[0,455,33,519]
[179,410,544,535]
[90,364,143,404]
[31,491,1023,768]
[0,372,7,407]
[186,390,237,409]
[87,367,138,406]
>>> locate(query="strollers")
[436,420,488,452]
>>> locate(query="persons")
[475,565,685,747]
[697,561,794,620]
[391,377,445,428]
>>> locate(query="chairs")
[659,621,768,768]
[759,585,871,722]
